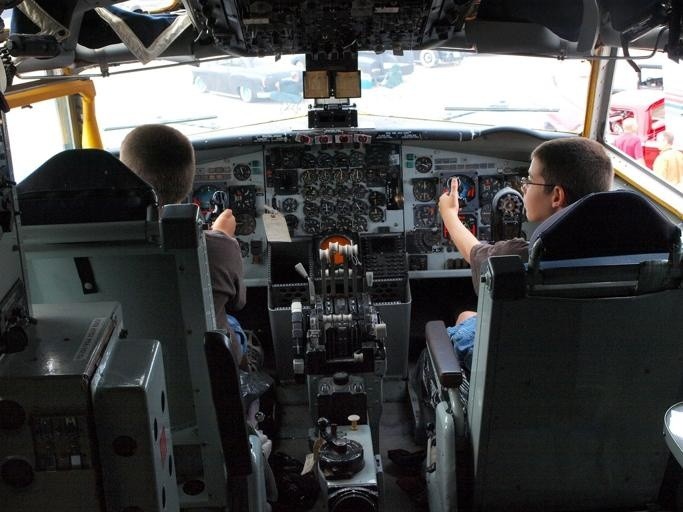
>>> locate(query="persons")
[438,138,614,382]
[611,118,642,160]
[653,132,683,183]
[120,124,247,365]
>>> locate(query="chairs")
[17,150,279,510]
[406,189,683,509]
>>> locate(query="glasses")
[519,176,553,194]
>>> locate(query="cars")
[184,49,480,105]
[608,86,665,145]
